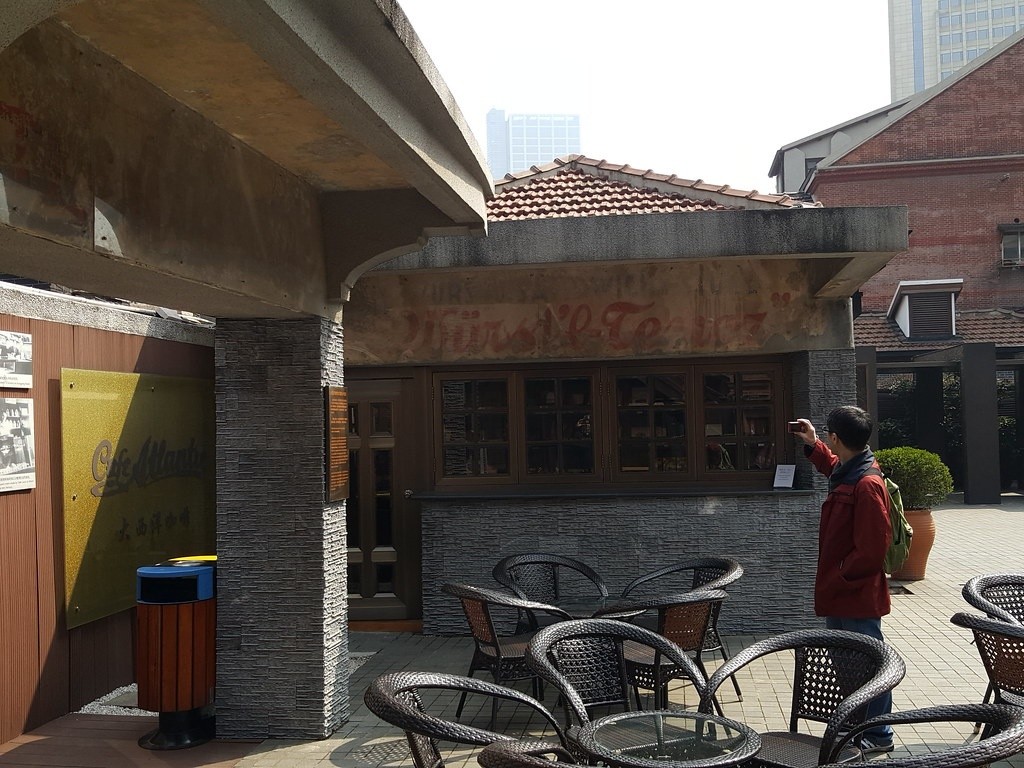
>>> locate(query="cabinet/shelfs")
[436,358,785,482]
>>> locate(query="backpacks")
[858,467,913,574]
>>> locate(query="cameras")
[787,422,806,434]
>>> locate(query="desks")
[577,710,763,768]
[539,594,650,709]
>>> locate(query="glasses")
[821,425,839,438]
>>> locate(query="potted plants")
[872,442,956,582]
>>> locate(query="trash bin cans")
[135,556,217,751]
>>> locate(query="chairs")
[696,626,909,768]
[491,550,609,699]
[953,608,1024,705]
[477,738,591,768]
[817,700,1024,768]
[364,670,568,768]
[439,577,575,732]
[962,573,1024,732]
[524,616,716,761]
[595,588,731,738]
[621,554,746,702]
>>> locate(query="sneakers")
[847,736,894,753]
[837,719,861,736]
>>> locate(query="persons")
[792,404,893,754]
